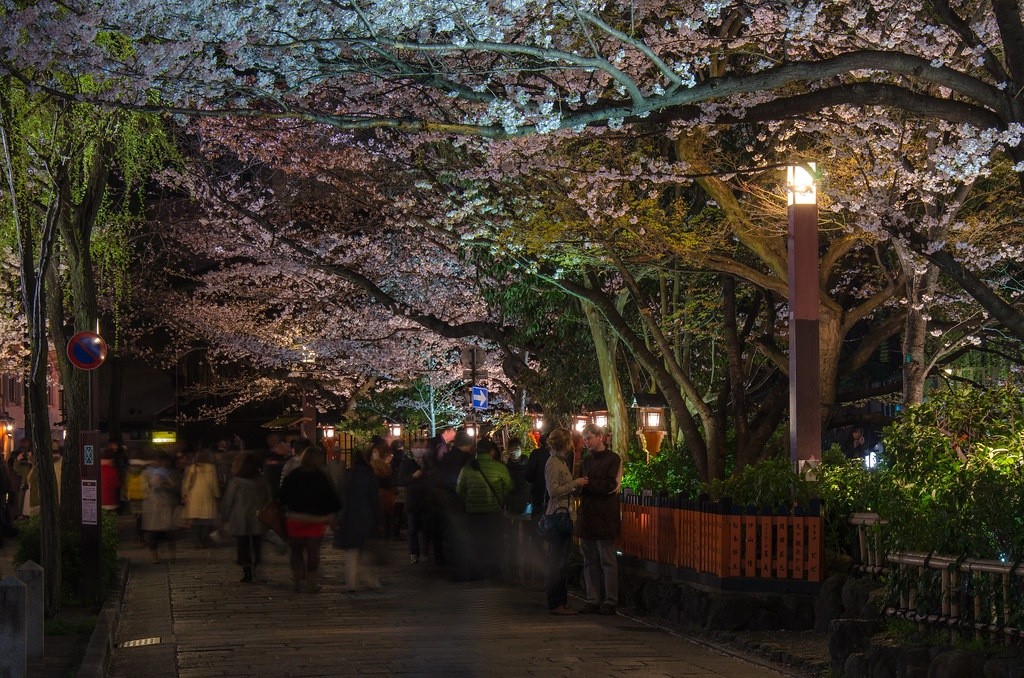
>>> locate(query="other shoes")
[563,603,579,614]
[548,605,575,616]
[597,604,616,615]
[582,603,600,614]
[240,575,257,585]
[484,568,493,578]
[410,554,419,563]
[458,571,482,581]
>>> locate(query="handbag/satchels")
[537,506,574,544]
[126,471,158,500]
[172,506,193,528]
[499,509,513,529]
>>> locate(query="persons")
[846,427,866,458]
[0,425,620,618]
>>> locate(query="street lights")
[568,416,589,477]
[175,343,210,417]
[783,159,822,496]
[636,408,667,464]
[530,414,543,446]
[322,426,337,460]
